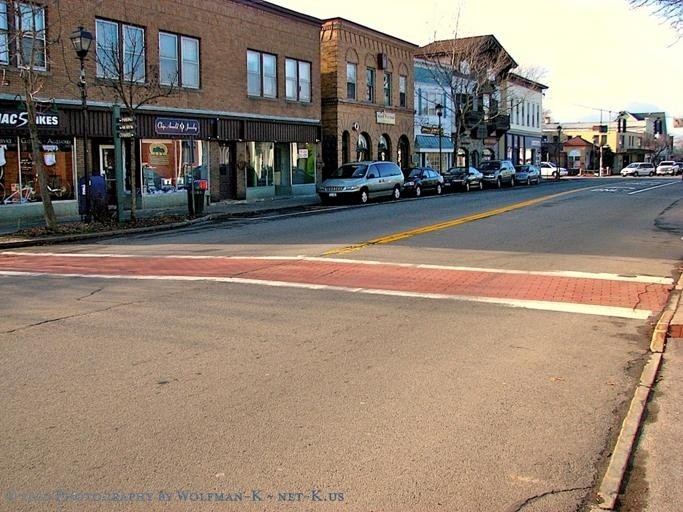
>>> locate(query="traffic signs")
[117,131,137,139]
[116,116,137,123]
[114,124,138,131]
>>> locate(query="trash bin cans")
[187,188,205,217]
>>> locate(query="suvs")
[182,161,260,196]
[314,157,406,205]
[474,159,517,189]
[655,161,680,177]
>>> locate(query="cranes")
[576,102,618,122]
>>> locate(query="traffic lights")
[658,120,663,135]
[652,120,658,135]
[623,118,627,133]
[617,118,621,133]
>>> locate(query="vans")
[620,161,656,177]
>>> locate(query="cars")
[512,164,542,186]
[439,166,485,191]
[541,161,569,177]
[676,162,683,172]
[401,164,445,197]
[257,164,314,185]
[143,168,163,193]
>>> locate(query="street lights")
[556,125,562,181]
[66,22,105,227]
[433,101,446,174]
